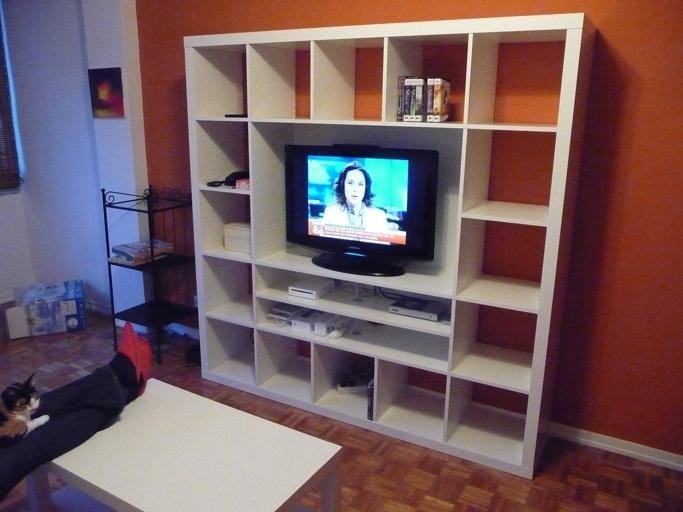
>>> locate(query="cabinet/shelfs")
[182,12,598,481]
[101,185,192,364]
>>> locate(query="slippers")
[119,320,153,396]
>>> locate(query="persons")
[1,321,154,506]
[318,160,392,249]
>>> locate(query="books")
[396,73,452,123]
[108,253,170,267]
[366,379,375,422]
[111,237,175,260]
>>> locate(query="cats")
[1,370,50,446]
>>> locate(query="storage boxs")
[6,279,87,340]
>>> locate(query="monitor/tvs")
[282,143,439,277]
